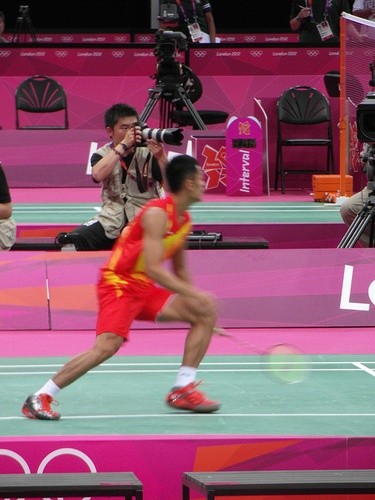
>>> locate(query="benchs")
[0,472,143,500]
[182,470,375,500]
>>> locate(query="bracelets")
[120,143,128,150]
[112,148,123,157]
[296,15,302,23]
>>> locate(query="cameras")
[130,121,184,147]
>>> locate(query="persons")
[21,156,222,420]
[0,163,16,251]
[66,102,170,251]
[352,0,375,40]
[289,0,351,47]
[160,0,216,44]
[339,186,375,248]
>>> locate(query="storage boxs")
[311,175,353,202]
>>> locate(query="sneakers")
[21,393,61,420]
[166,383,220,412]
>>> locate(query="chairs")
[274,85,335,194]
[15,74,69,130]
[169,63,229,130]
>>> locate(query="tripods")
[11,5,37,43]
[137,81,208,130]
